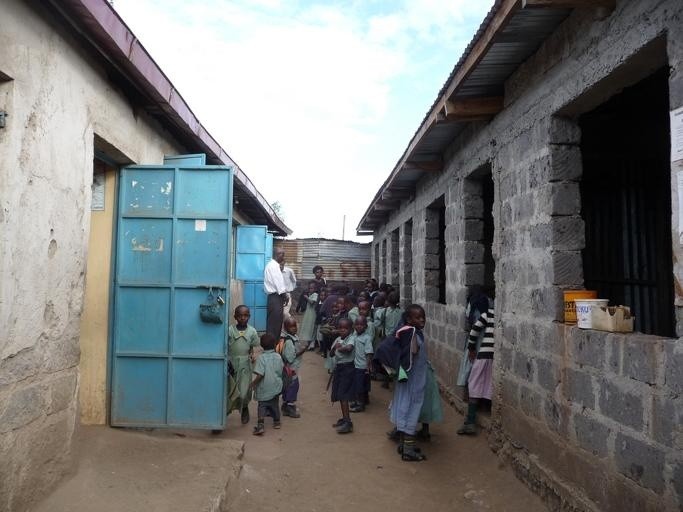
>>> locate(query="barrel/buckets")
[574,298,609,330]
[563,290,599,327]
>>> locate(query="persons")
[354,301,375,337]
[282,318,300,418]
[386,304,427,461]
[366,276,394,296]
[373,296,385,327]
[386,291,398,307]
[299,282,318,352]
[251,333,282,435]
[328,319,354,434]
[212,305,260,436]
[358,291,368,303]
[312,265,325,288]
[456,290,495,435]
[263,247,286,336]
[280,260,297,317]
[349,315,374,412]
[386,366,442,443]
[318,284,353,319]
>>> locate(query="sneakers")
[349,407,366,413]
[305,347,314,352]
[283,406,302,419]
[332,418,350,426]
[398,445,420,454]
[404,454,426,462]
[338,421,353,433]
[384,431,403,441]
[350,402,361,407]
[319,351,328,358]
[456,421,476,435]
[416,432,432,442]
[281,402,298,412]
[273,419,280,428]
[252,422,264,436]
[240,409,250,425]
